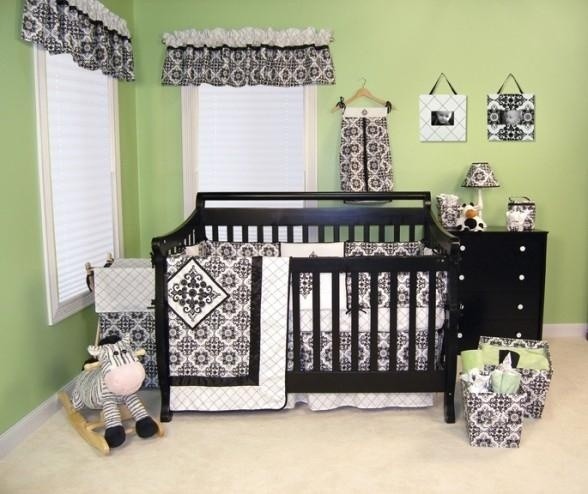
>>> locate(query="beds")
[150,191,465,423]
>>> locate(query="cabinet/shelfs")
[450,227,549,350]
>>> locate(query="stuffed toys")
[456,203,484,232]
[70,336,158,450]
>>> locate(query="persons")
[505,110,520,125]
[434,111,452,125]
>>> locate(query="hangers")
[329,76,399,117]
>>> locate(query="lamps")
[461,162,501,219]
